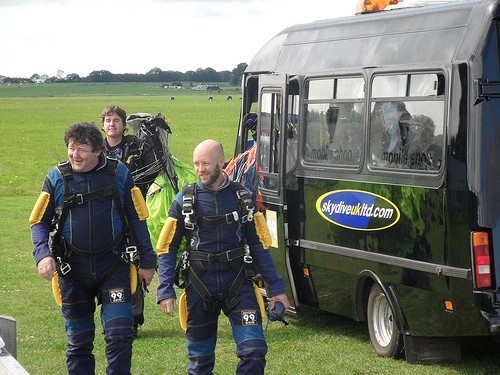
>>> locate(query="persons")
[368,185,427,257]
[30,122,158,375]
[99,105,158,201]
[154,139,289,375]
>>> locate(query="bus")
[233,0,500,364]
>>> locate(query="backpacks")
[124,111,171,178]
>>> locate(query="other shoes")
[131,323,139,336]
